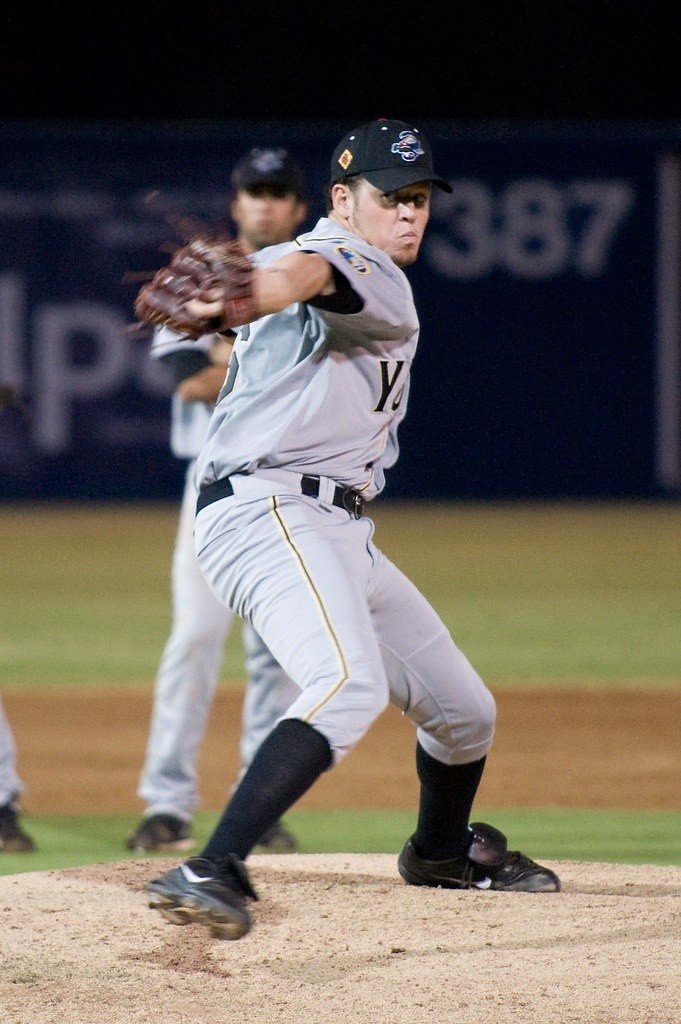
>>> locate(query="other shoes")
[126,815,195,853]
[0,806,36,853]
[255,824,298,853]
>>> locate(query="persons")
[134,120,561,941]
[127,154,309,854]
[0,706,36,852]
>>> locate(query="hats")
[330,117,454,196]
[231,147,307,192]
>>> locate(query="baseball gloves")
[133,236,260,337]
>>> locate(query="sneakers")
[397,822,561,894]
[144,851,257,940]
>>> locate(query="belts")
[195,476,364,518]
